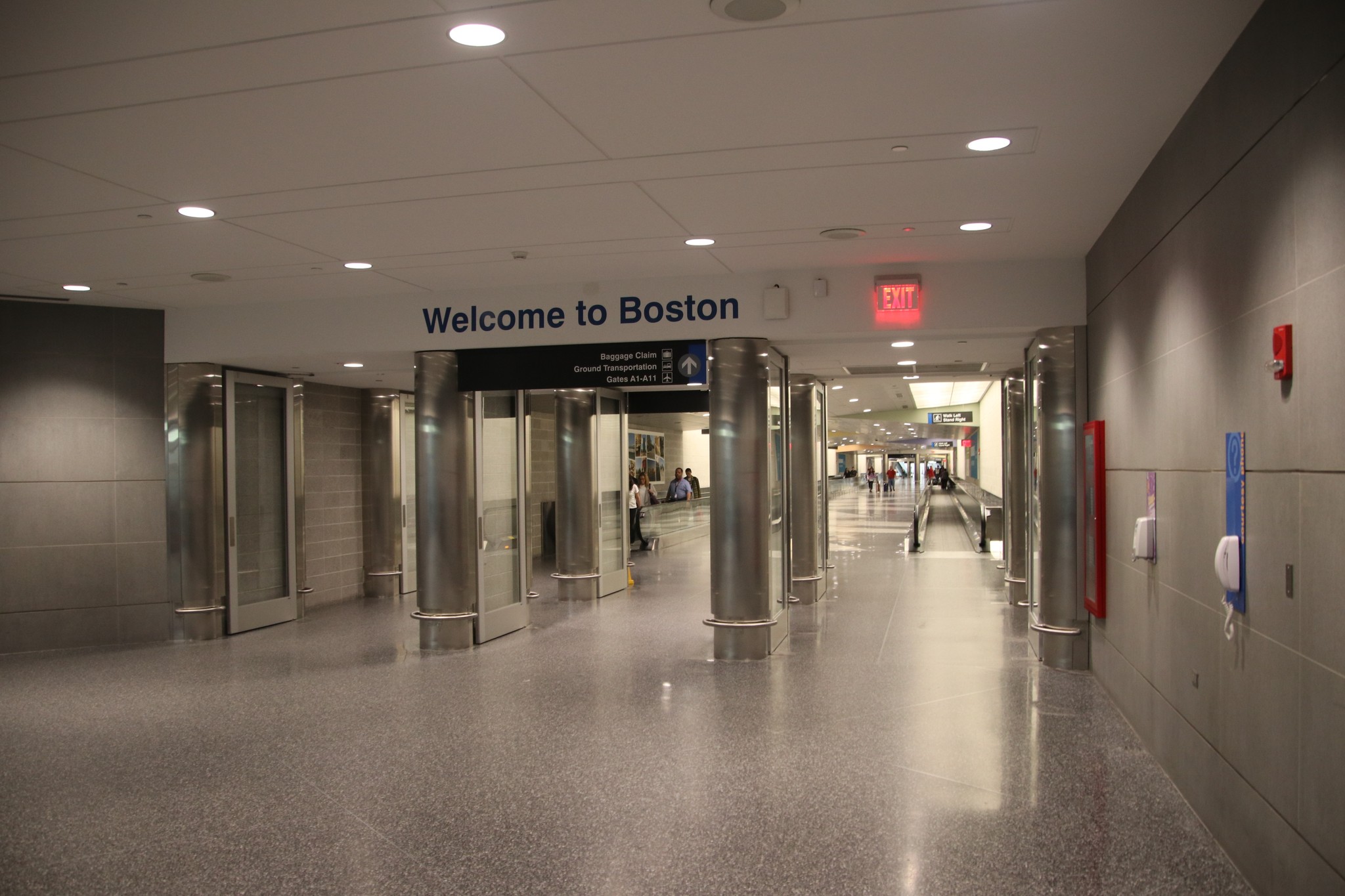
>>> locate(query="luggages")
[883,482,889,492]
[876,476,881,493]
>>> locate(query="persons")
[926,465,949,490]
[629,472,657,545]
[844,466,878,493]
[886,466,896,492]
[683,468,701,500]
[902,468,905,479]
[665,468,692,503]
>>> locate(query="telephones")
[1214,535,1240,592]
[1131,516,1154,561]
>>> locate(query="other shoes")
[631,542,635,545]
[890,490,892,491]
[893,489,895,491]
[870,490,872,493]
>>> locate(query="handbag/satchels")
[649,483,659,505]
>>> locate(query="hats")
[868,466,874,470]
